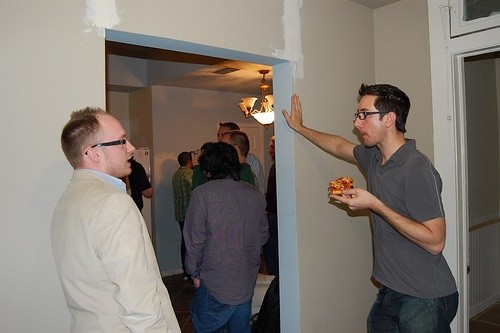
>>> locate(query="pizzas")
[328,177,354,195]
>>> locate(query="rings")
[195,153,198,155]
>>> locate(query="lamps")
[236,69,275,130]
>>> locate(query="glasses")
[354,111,389,120]
[84,138,128,156]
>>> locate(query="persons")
[216,121,268,205]
[190,130,255,193]
[201,142,215,153]
[119,140,154,216]
[282,81,459,333]
[182,141,268,333]
[172,152,194,285]
[263,135,280,317]
[49,106,183,333]
